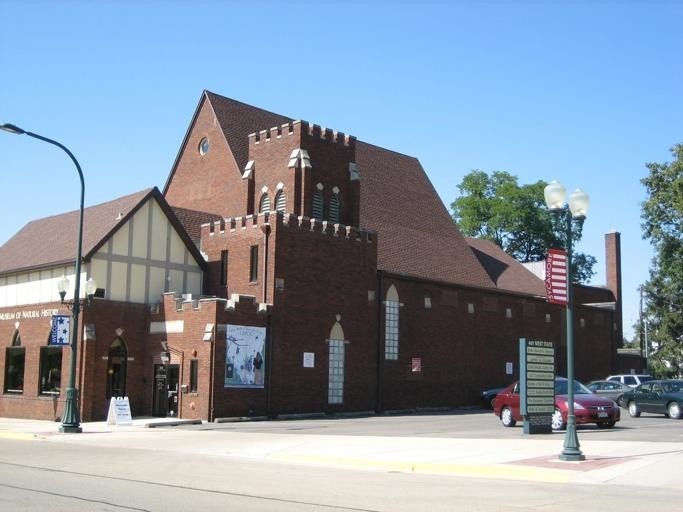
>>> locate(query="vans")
[606,373,650,389]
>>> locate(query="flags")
[544,248,568,306]
[50,315,70,345]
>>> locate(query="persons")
[252,352,263,385]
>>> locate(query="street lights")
[1,123,85,433]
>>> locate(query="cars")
[585,381,633,401]
[479,387,507,408]
[617,379,682,421]
[494,376,620,429]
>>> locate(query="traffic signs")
[540,181,590,463]
